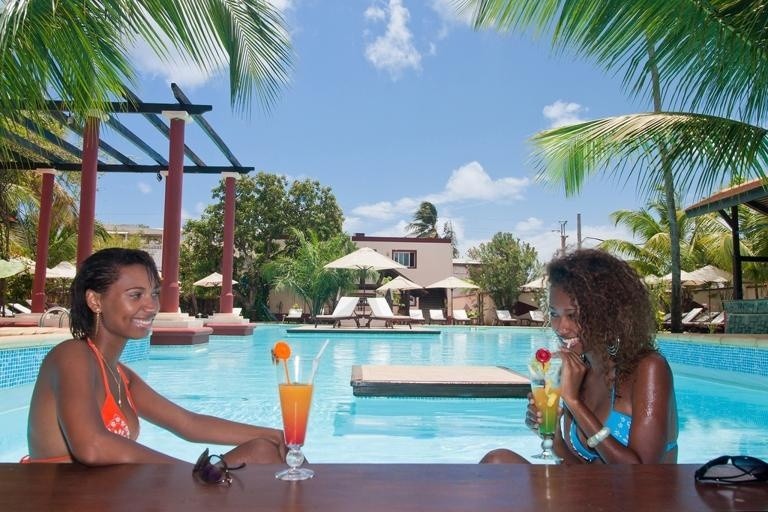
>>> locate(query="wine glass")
[272,355,319,482]
[527,363,566,461]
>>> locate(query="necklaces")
[98,355,126,411]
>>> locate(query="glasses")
[193,448,246,487]
[695,455,767,485]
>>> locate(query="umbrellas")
[7,253,50,278]
[424,276,479,316]
[44,260,77,305]
[319,246,407,315]
[518,275,551,291]
[374,274,425,293]
[637,264,733,321]
[189,271,238,310]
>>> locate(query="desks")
[1,463,768,512]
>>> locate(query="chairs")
[659,307,726,334]
[282,295,545,328]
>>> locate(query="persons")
[24,245,310,468]
[475,248,680,468]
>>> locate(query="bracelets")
[584,426,610,452]
